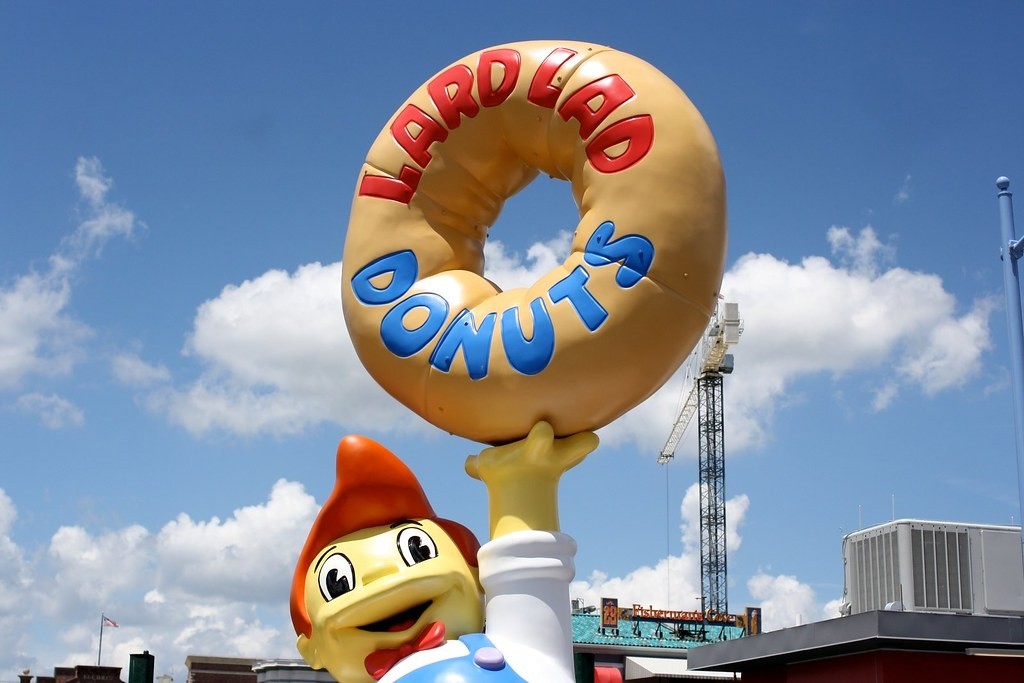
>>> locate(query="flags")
[103,616,119,628]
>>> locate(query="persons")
[286,419,602,683]
